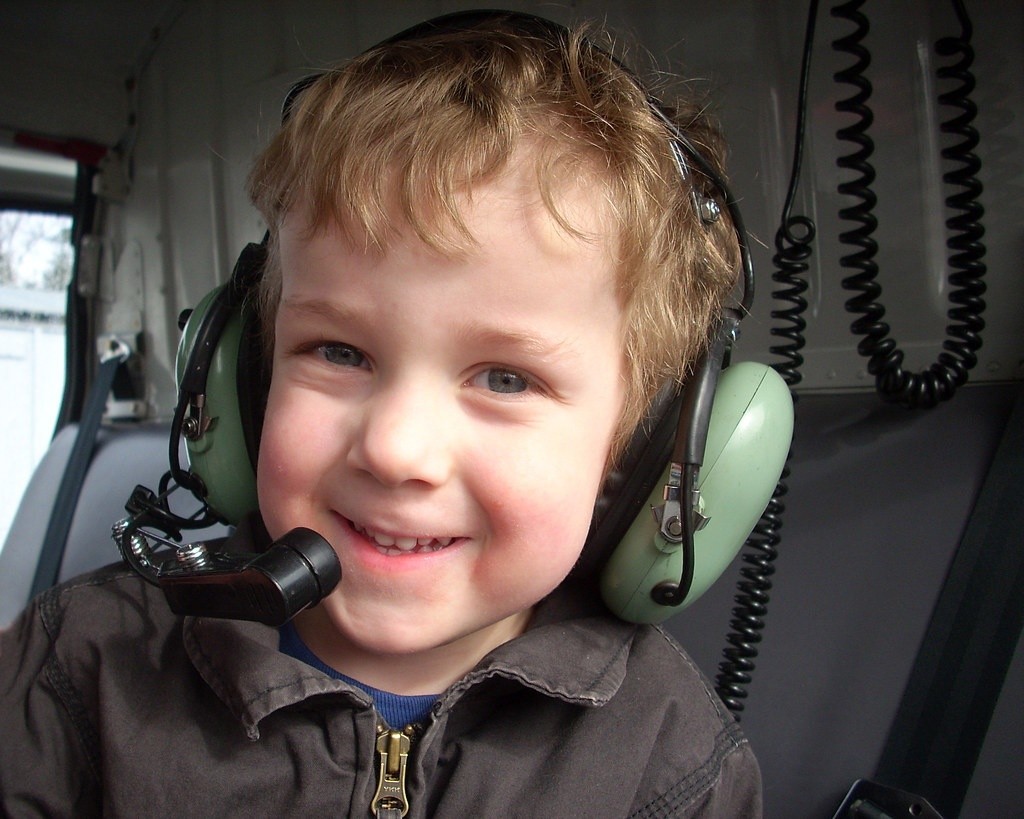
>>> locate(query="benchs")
[1,378,1024,819]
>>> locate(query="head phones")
[167,8,795,623]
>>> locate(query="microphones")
[111,469,341,627]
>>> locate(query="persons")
[0,16,760,819]
